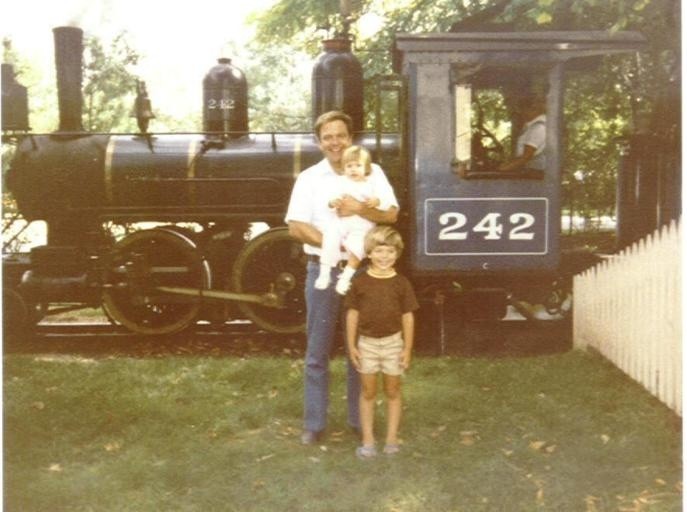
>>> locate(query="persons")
[310,145,385,297]
[282,109,402,447]
[338,222,420,461]
[490,96,545,174]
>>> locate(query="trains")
[2,22,652,347]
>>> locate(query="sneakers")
[300,430,319,444]
[349,423,401,460]
[314,273,350,296]
